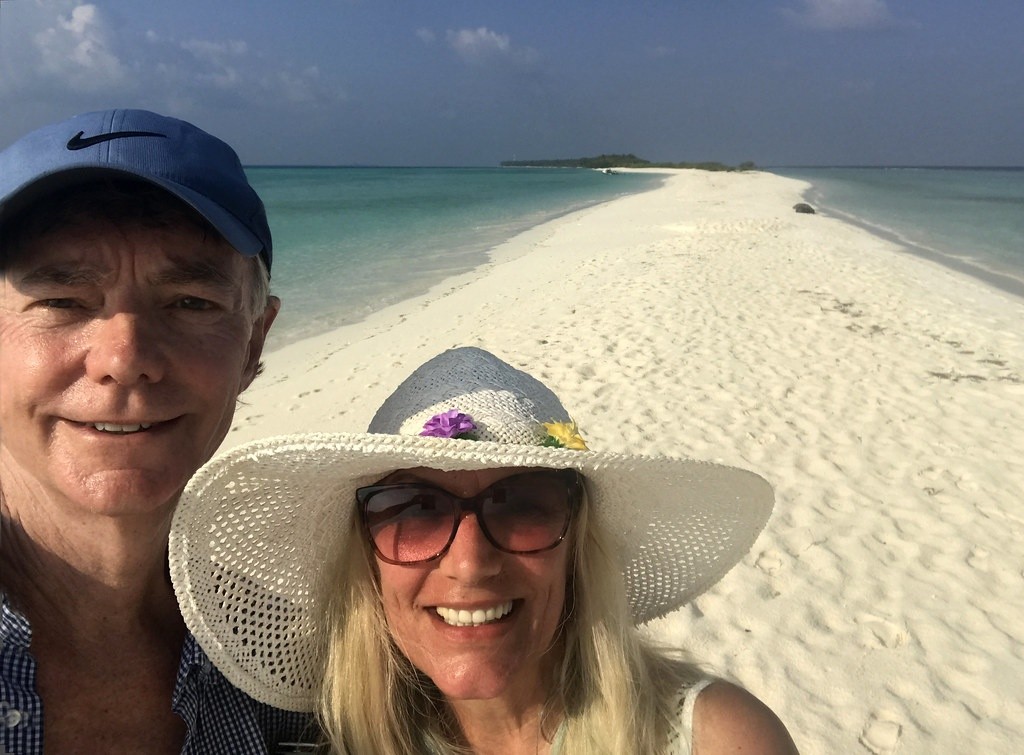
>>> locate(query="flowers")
[535,415,592,452]
[419,409,481,441]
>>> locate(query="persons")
[168,346,799,755]
[0,108,332,755]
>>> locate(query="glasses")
[355,468,585,567]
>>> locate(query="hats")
[1,107,274,278]
[165,345,776,714]
